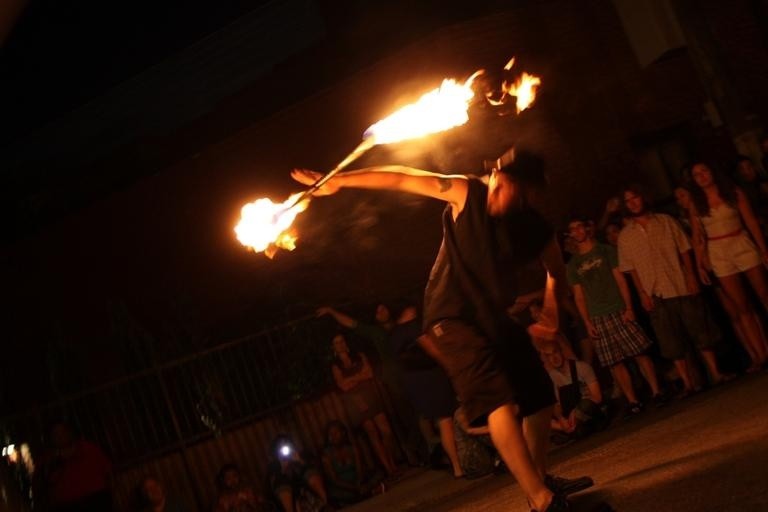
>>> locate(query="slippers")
[648,392,669,404]
[626,402,643,416]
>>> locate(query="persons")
[128,419,384,511]
[292,143,612,511]
[313,299,509,479]
[505,160,763,434]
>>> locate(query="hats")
[481,148,543,185]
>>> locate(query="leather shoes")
[525,475,597,512]
